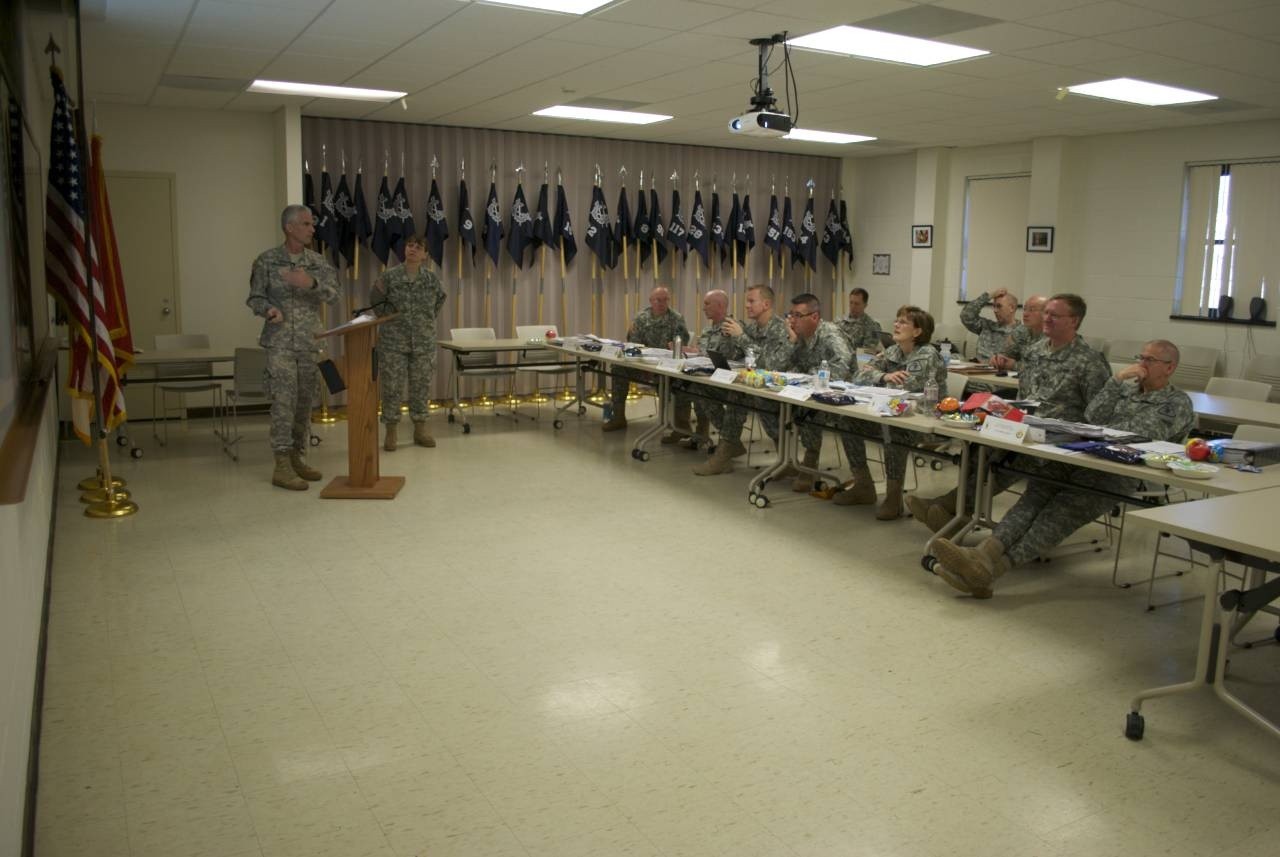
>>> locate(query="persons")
[660,288,753,452]
[599,285,691,431]
[368,236,448,451]
[959,289,1024,401]
[692,283,797,475]
[906,292,1114,545]
[774,292,861,492]
[244,204,344,490]
[988,293,1062,402]
[831,288,883,373]
[832,306,948,521]
[928,339,1194,600]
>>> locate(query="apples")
[1188,439,1210,462]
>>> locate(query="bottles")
[923,372,940,418]
[817,359,830,390]
[746,348,756,375]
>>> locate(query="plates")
[1166,460,1219,479]
[1139,453,1189,469]
[942,413,978,428]
[764,383,785,392]
[625,357,659,361]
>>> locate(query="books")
[1202,438,1280,468]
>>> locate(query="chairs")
[220,347,273,462]
[150,332,229,448]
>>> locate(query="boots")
[601,402,627,432]
[691,438,735,475]
[832,464,876,504]
[660,399,694,444]
[790,448,818,490]
[935,553,1012,594]
[291,456,321,481]
[731,438,747,457]
[933,534,1005,590]
[383,423,399,451]
[695,412,709,438]
[875,477,906,520]
[272,455,309,490]
[414,422,436,447]
[926,501,967,540]
[907,486,959,527]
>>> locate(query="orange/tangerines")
[939,397,961,412]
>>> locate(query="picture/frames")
[912,225,933,248]
[872,253,891,274]
[1025,226,1054,253]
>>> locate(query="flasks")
[672,335,682,361]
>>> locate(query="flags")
[301,165,858,274]
[43,67,134,449]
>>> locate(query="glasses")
[1134,354,1171,365]
[787,311,816,319]
[1041,310,1075,320]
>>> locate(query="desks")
[437,322,1280,745]
[113,347,323,458]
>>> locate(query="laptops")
[706,349,731,370]
